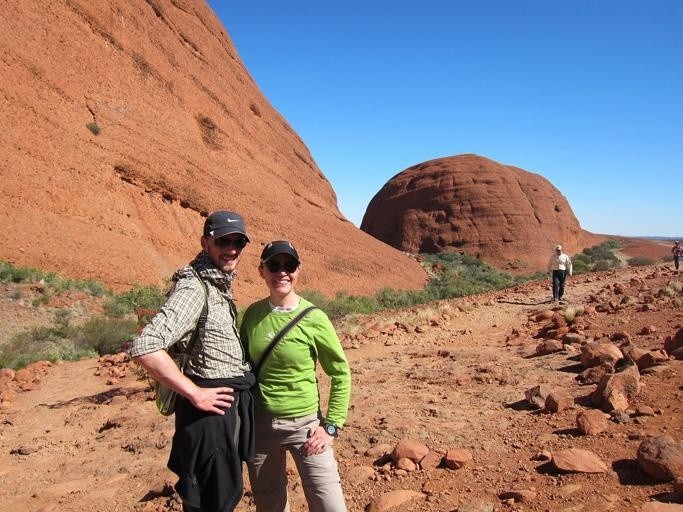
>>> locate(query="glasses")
[213,236,248,248]
[263,260,299,273]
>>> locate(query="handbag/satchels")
[153,277,210,416]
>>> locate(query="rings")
[316,444,320,449]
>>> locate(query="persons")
[670,240,683,271]
[240,240,350,511]
[125,211,256,512]
[545,244,572,302]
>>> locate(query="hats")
[260,240,301,265]
[203,209,251,244]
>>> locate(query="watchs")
[322,421,337,436]
